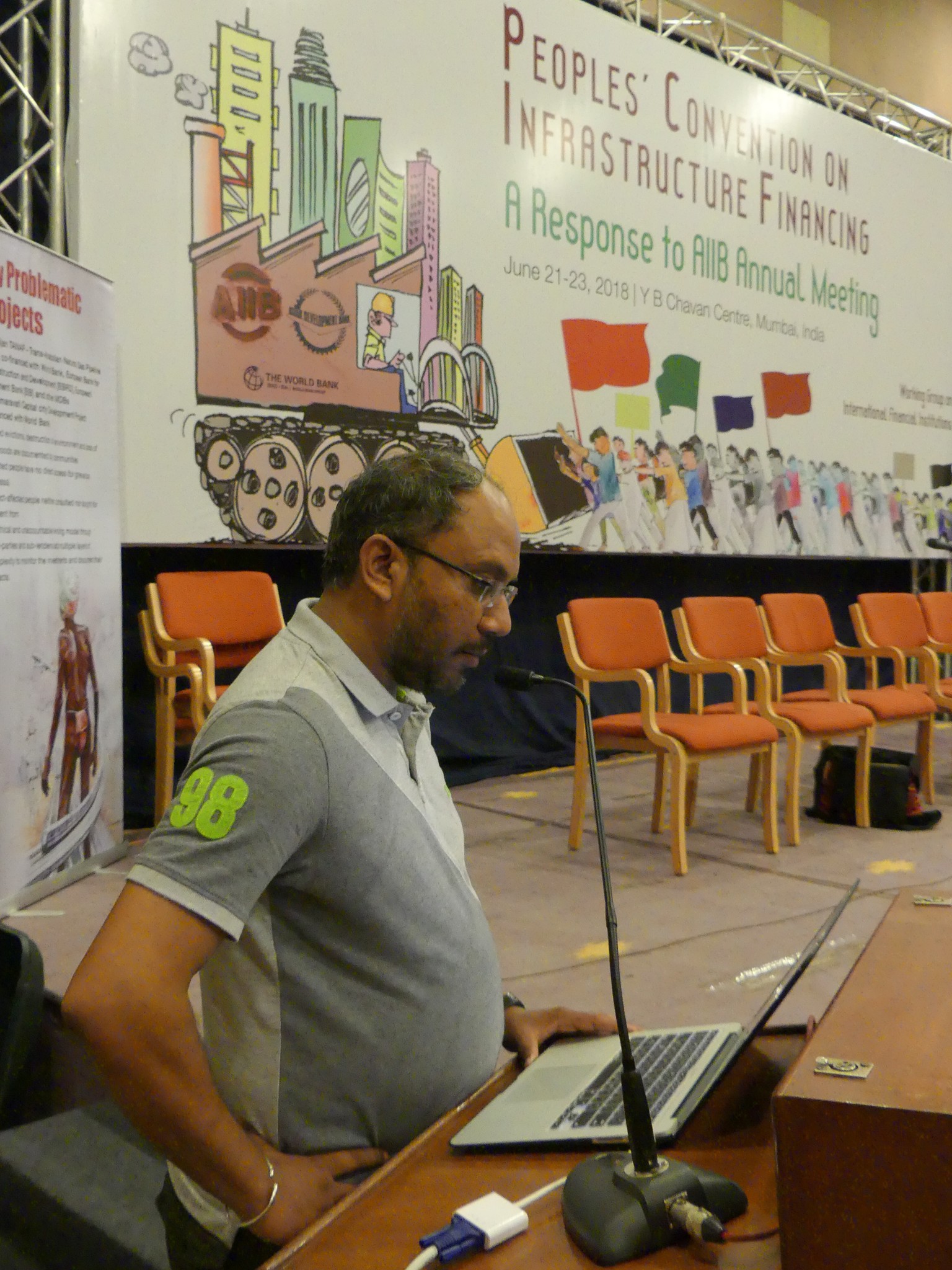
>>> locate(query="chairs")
[554,591,952,877]
[141,570,285,829]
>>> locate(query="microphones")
[494,663,749,1265]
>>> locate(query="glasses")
[357,530,516,611]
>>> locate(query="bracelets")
[502,992,528,1052]
[225,1149,279,1227]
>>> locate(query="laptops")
[449,878,862,1150]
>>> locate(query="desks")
[774,884,952,1270]
[258,1025,813,1270]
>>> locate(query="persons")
[61,453,640,1270]
